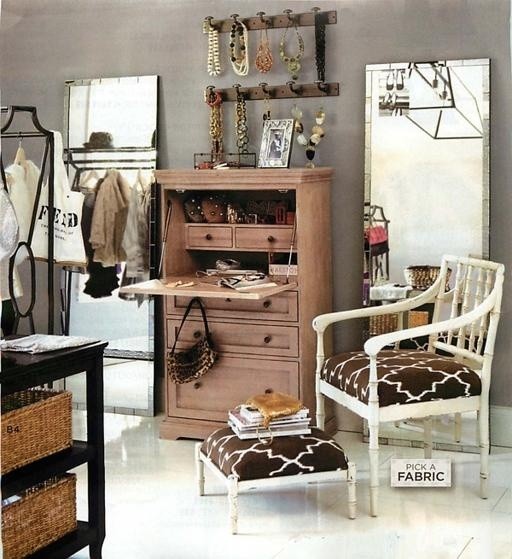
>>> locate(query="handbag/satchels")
[245,391,303,445]
[364,216,389,259]
[25,130,87,265]
[166,297,218,385]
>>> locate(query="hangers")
[4,130,41,186]
[79,159,156,193]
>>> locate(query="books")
[207,269,258,275]
[228,404,312,440]
[199,271,278,291]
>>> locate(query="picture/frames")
[257,119,296,168]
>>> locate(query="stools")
[195,428,358,535]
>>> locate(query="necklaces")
[204,19,326,169]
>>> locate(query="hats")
[83,132,113,148]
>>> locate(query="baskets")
[2,474,76,555]
[369,311,428,335]
[403,265,451,289]
[1,388,73,475]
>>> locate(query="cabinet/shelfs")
[155,166,338,441]
[1,331,109,558]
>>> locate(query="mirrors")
[359,57,492,455]
[57,73,161,417]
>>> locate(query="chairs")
[313,253,506,518]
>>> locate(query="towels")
[1,333,101,355]
[369,279,413,301]
